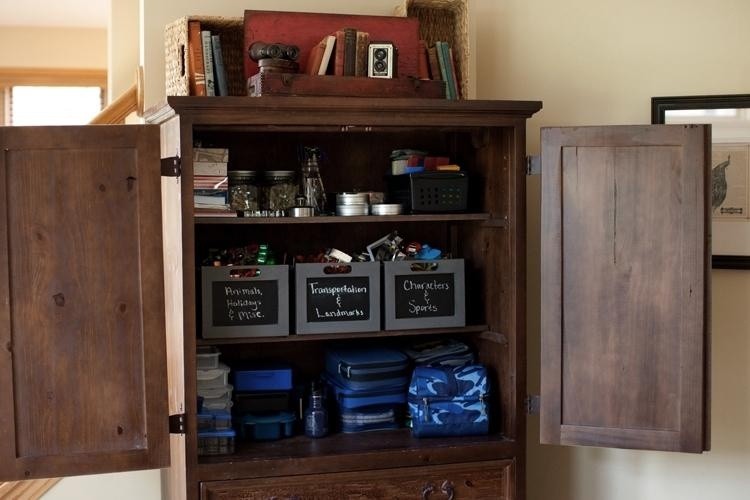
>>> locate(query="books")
[308,26,369,77]
[418,40,461,101]
[192,147,239,218]
[186,20,231,98]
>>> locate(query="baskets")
[388,174,468,212]
[393,0,471,100]
[165,15,244,96]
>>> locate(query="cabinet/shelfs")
[3,96,713,500]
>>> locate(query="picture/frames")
[651,94,750,271]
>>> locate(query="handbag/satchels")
[407,365,491,437]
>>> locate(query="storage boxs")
[385,259,474,331]
[199,262,290,339]
[295,260,382,335]
